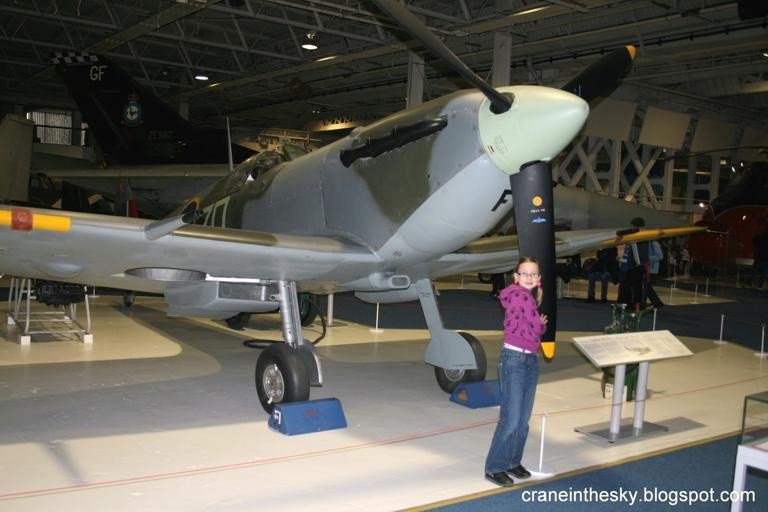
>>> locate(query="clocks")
[120,94,146,126]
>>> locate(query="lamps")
[194,70,211,81]
[302,27,319,51]
[763,50,768,57]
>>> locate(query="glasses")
[515,271,539,277]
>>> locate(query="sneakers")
[485,465,531,486]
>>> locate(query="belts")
[503,343,532,354]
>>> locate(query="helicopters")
[662,144,767,280]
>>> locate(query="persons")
[484,256,549,487]
[584,217,690,316]
